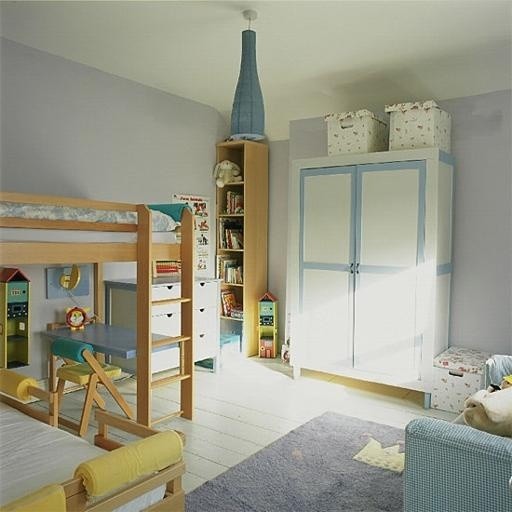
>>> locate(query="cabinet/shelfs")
[1,268,31,370]
[289,149,454,409]
[215,141,269,357]
[104,277,223,379]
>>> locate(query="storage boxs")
[384,101,452,154]
[323,109,390,156]
[430,345,493,414]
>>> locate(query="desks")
[40,323,179,360]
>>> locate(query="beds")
[0,370,186,512]
[1,193,195,428]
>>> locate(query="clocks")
[65,307,90,331]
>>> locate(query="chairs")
[404,355,512,512]
[47,338,133,437]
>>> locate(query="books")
[215,190,244,319]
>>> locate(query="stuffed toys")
[212,161,244,188]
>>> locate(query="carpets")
[185,411,406,512]
